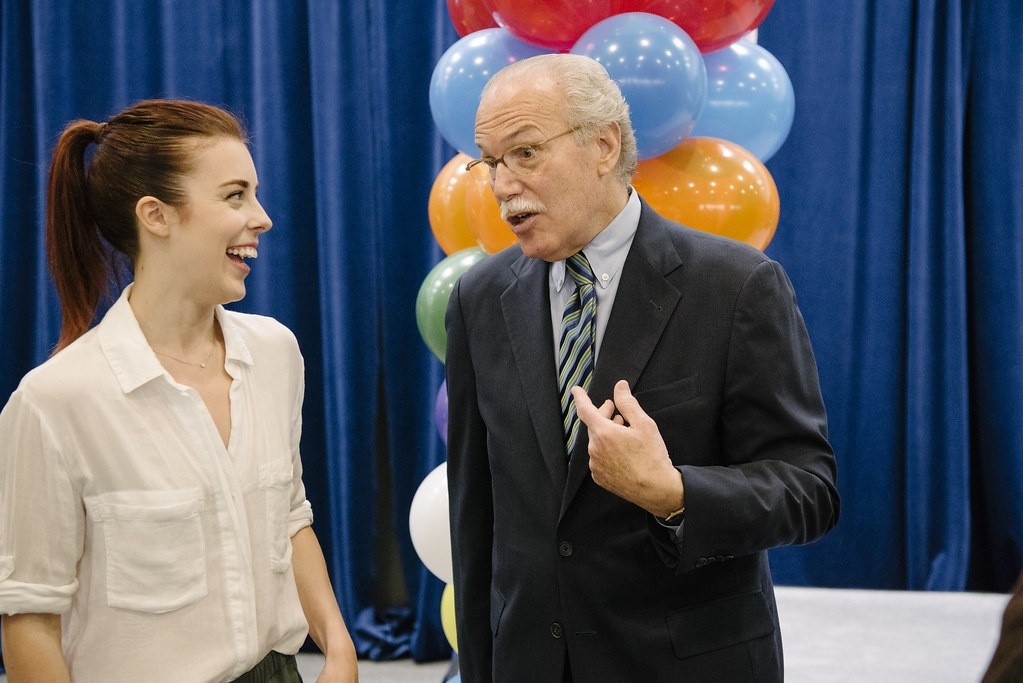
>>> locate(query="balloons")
[408,0,795,656]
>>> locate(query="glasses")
[465,123,584,184]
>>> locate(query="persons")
[0,99,359,683]
[445,54,841,683]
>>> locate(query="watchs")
[659,506,686,526]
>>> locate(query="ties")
[555,251,597,463]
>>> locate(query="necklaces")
[152,327,215,368]
